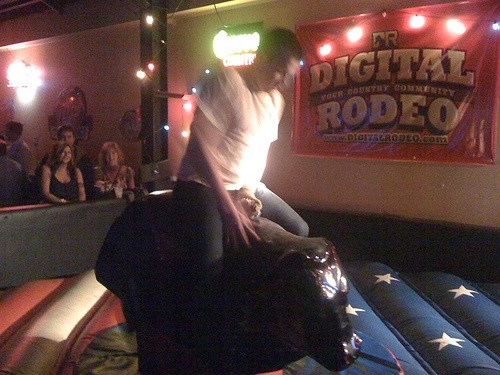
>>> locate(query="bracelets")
[59,198,65,204]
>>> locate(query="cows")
[95,194,362,374]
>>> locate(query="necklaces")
[54,173,60,183]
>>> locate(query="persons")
[0,121,135,206]
[174,27,308,291]
